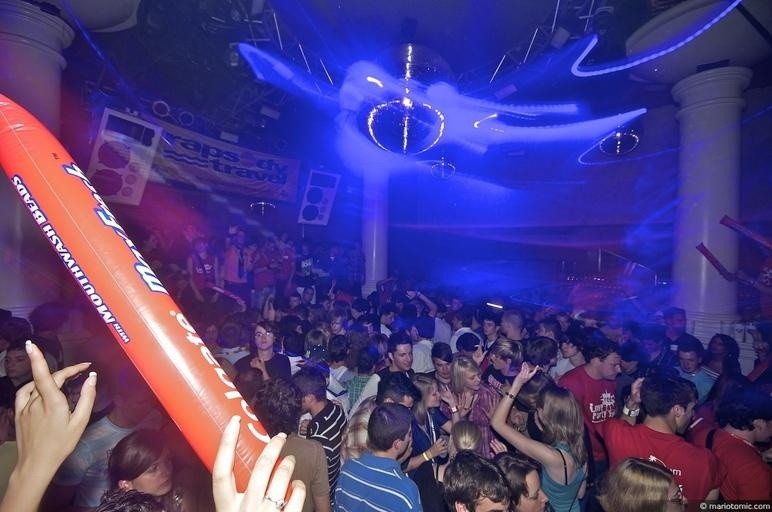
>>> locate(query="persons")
[0,222,772,512]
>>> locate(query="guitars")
[193,274,240,301]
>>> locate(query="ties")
[237,246,245,279]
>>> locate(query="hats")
[743,384,771,410]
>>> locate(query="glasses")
[255,333,273,337]
[668,484,684,504]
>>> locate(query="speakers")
[295,168,341,226]
[81,105,163,207]
